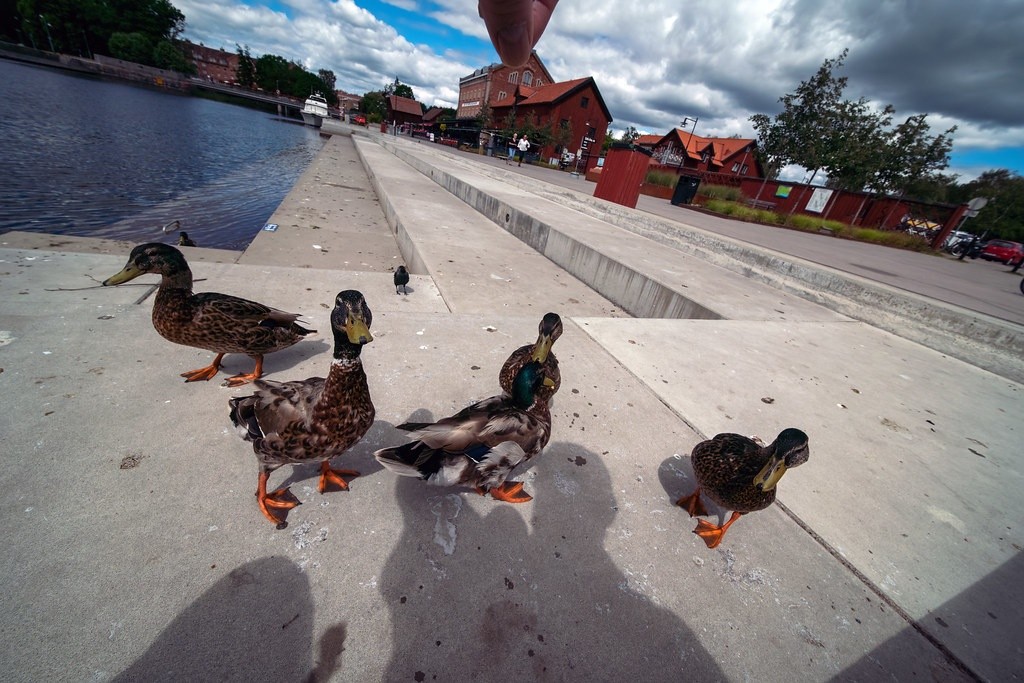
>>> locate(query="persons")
[477,0,559,70]
[506,133,519,166]
[517,134,531,166]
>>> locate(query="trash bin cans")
[593,141,654,208]
[670,174,701,206]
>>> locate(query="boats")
[300,88,334,127]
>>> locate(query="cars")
[899,211,1024,265]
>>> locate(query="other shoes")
[518,164,521,167]
[506,158,508,163]
[509,162,511,165]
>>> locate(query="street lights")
[675,116,700,174]
[389,88,398,136]
[582,118,599,175]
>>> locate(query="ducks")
[499,312,564,399]
[674,426,810,548]
[372,361,556,502]
[102,242,317,388]
[393,266,410,296]
[177,231,195,247]
[226,289,378,525]
